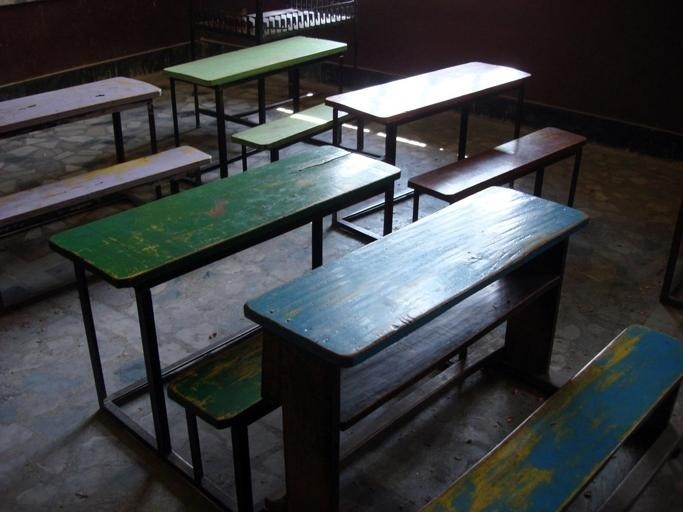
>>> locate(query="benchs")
[407,125,587,224]
[0,146,210,246]
[231,104,349,171]
[167,324,283,510]
[415,323,683,512]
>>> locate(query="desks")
[0,78,160,205]
[240,186,587,511]
[160,33,349,186]
[324,62,531,240]
[46,145,397,458]
